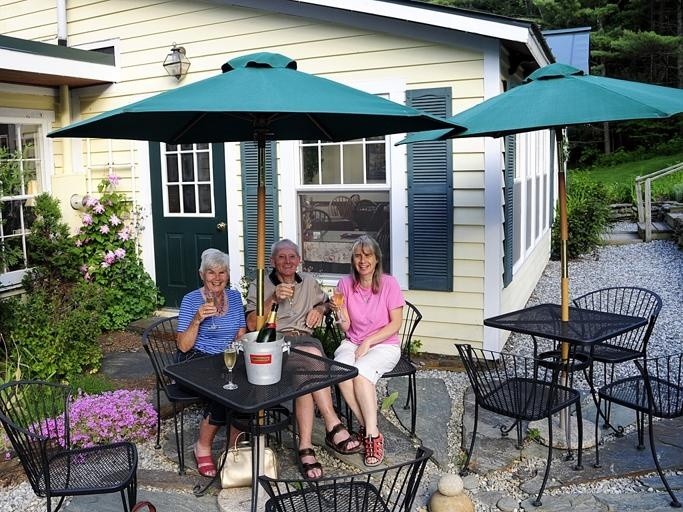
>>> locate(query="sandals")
[364,432,385,466]
[357,426,367,456]
[324,423,364,456]
[296,446,324,480]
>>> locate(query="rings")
[203,311,205,314]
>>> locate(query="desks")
[161,348,359,504]
[482,303,648,461]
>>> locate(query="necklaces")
[207,291,224,315]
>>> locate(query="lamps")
[161,41,192,81]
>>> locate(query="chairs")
[564,285,660,454]
[141,315,206,478]
[0,380,140,512]
[300,194,388,274]
[325,297,422,440]
[254,447,434,512]
[593,350,681,507]
[454,343,583,507]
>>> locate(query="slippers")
[194,446,216,478]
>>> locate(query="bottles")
[256,301,281,344]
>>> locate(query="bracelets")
[272,290,276,301]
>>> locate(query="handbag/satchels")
[217,432,278,489]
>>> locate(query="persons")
[176,248,246,477]
[331,234,406,468]
[246,239,364,481]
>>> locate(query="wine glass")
[222,343,238,390]
[284,284,296,316]
[329,286,346,325]
[205,291,222,331]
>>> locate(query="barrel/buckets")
[238,332,291,386]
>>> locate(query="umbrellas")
[46,52,440,512]
[393,62,682,451]
